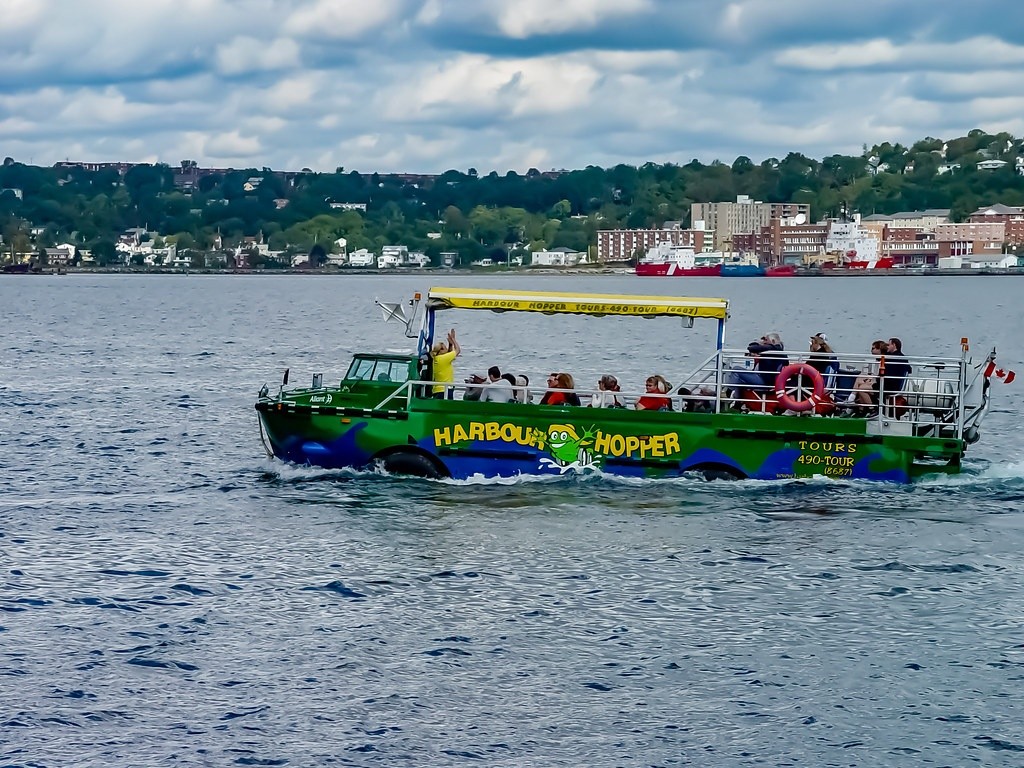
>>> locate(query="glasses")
[554,378,559,381]
[598,380,602,384]
[546,380,553,383]
[871,347,876,350]
[646,384,653,387]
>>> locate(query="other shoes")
[867,410,879,420]
[720,391,730,402]
[837,398,855,404]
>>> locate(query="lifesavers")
[775,363,824,412]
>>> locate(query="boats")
[256,287,997,485]
[636,259,822,278]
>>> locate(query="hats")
[470,370,487,381]
[809,333,828,341]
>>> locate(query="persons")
[430,328,461,400]
[461,332,911,419]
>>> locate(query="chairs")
[883,371,911,419]
[822,367,838,395]
